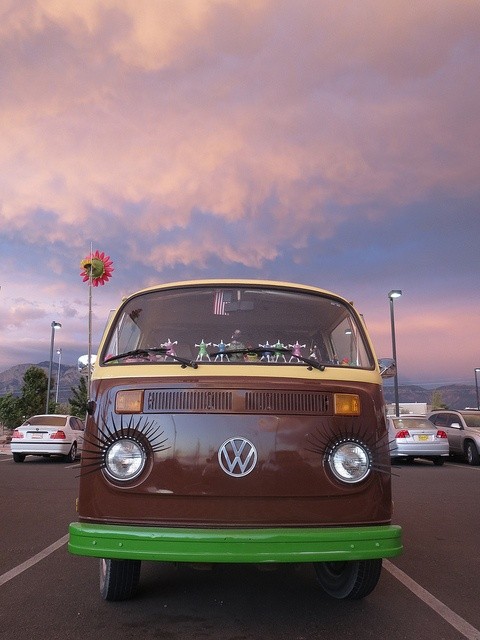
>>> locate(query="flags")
[211,290,233,317]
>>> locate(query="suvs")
[428,409,480,465]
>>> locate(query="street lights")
[56,348,62,403]
[388,290,402,417]
[474,368,480,410]
[44,321,61,414]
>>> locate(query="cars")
[10,414,85,463]
[387,416,449,466]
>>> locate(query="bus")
[68,242,403,601]
